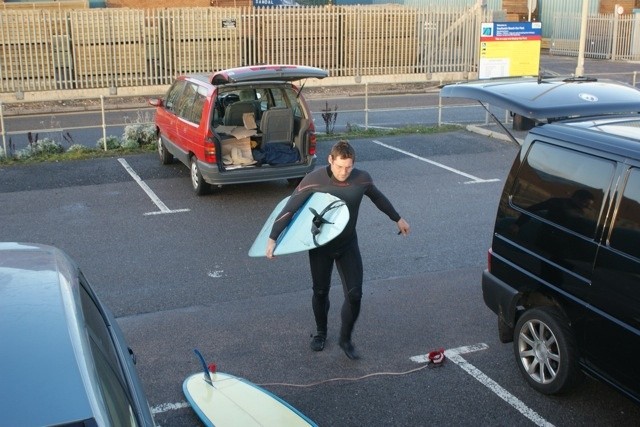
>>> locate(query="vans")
[439,75,640,401]
[0,240,156,425]
[149,64,328,194]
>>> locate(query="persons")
[266,139,410,361]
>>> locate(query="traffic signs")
[478,20,541,125]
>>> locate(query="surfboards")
[248,192,350,256]
[182,349,319,427]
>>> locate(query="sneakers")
[311,331,327,351]
[339,337,359,361]
